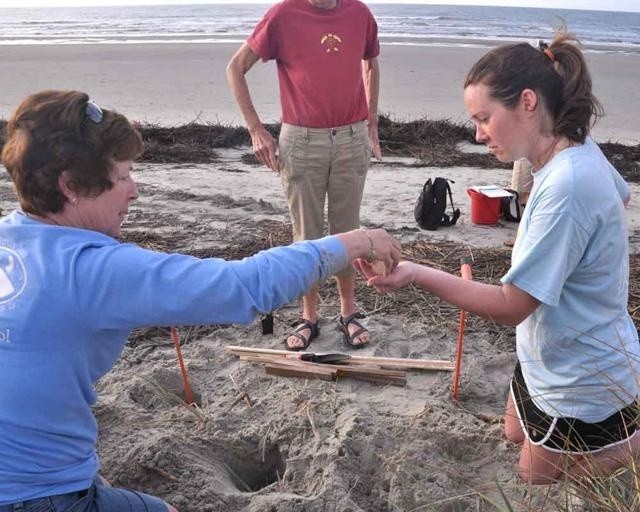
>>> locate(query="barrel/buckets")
[467,189,501,224]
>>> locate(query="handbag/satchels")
[501,189,520,222]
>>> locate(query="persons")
[0,87,401,512]
[225,0,383,353]
[352,31,640,487]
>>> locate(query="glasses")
[80,101,103,129]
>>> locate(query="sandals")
[337,314,370,348]
[286,318,318,351]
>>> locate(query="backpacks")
[414,178,460,230]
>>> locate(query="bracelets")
[366,233,377,264]
